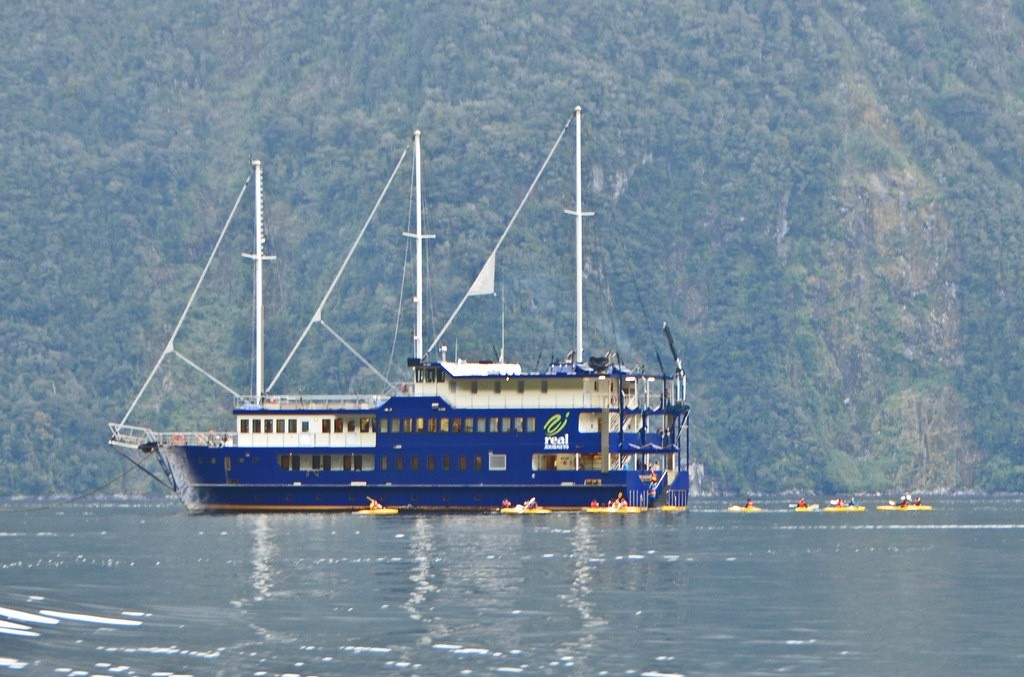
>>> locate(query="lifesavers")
[611,394,618,406]
[172,433,185,445]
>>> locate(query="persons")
[835,496,844,507]
[590,497,628,508]
[847,497,857,506]
[648,476,657,508]
[745,498,754,508]
[524,497,538,510]
[502,498,511,508]
[637,458,661,472]
[913,497,921,506]
[796,497,809,508]
[369,500,382,510]
[899,495,909,507]
[205,426,217,448]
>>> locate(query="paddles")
[617,491,623,500]
[524,497,536,508]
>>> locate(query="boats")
[581,506,646,513]
[728,505,760,512]
[795,504,866,512]
[876,505,932,511]
[352,510,398,515]
[496,507,551,514]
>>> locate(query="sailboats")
[108,106,692,512]
